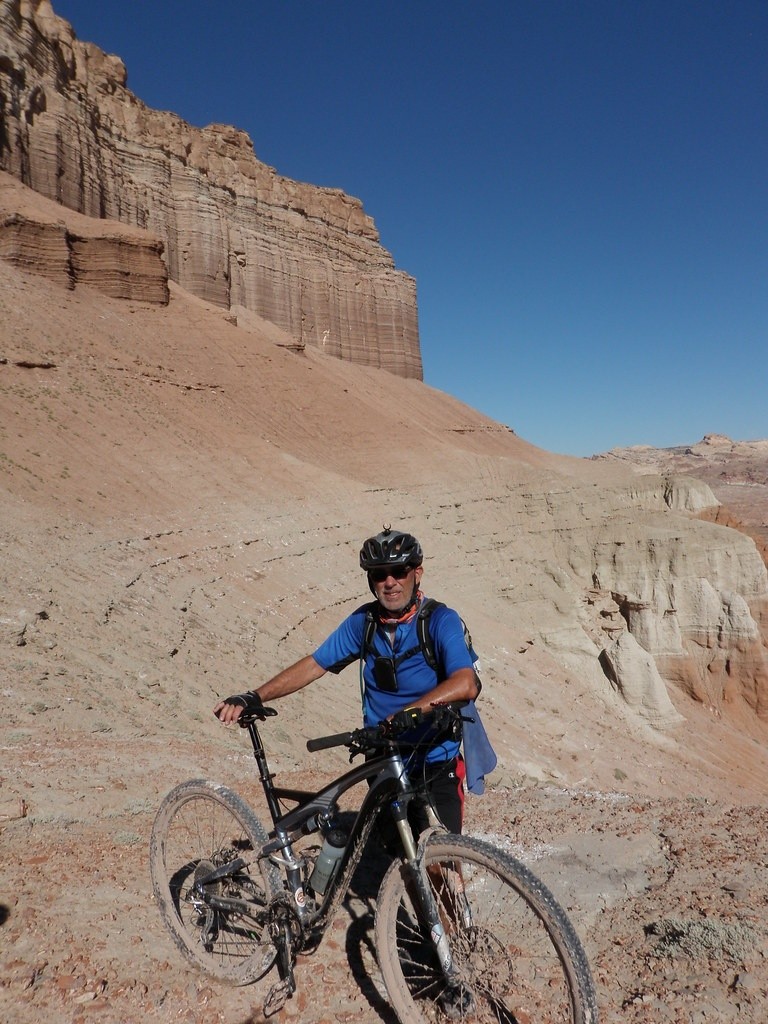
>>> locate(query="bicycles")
[151,699,600,1024]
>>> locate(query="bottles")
[309,832,349,896]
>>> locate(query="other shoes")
[437,981,477,1020]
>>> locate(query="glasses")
[368,564,413,583]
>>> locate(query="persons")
[213,523,482,1017]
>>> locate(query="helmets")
[359,523,422,571]
